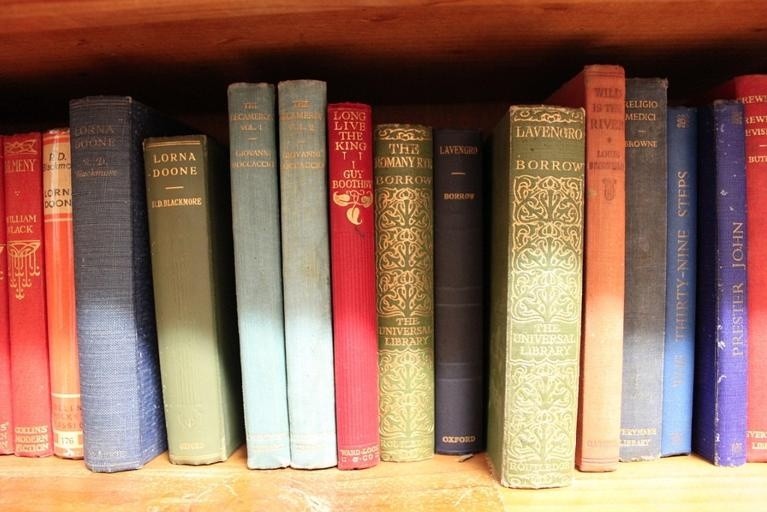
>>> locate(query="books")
[69,95,141,474]
[549,63,627,473]
[375,120,435,462]
[227,81,290,468]
[489,107,586,488]
[7,130,50,455]
[328,100,380,471]
[620,77,669,462]
[434,127,496,455]
[663,104,700,457]
[705,75,767,465]
[142,135,228,465]
[695,105,748,466]
[45,128,82,460]
[0,226,14,456]
[280,78,337,472]
